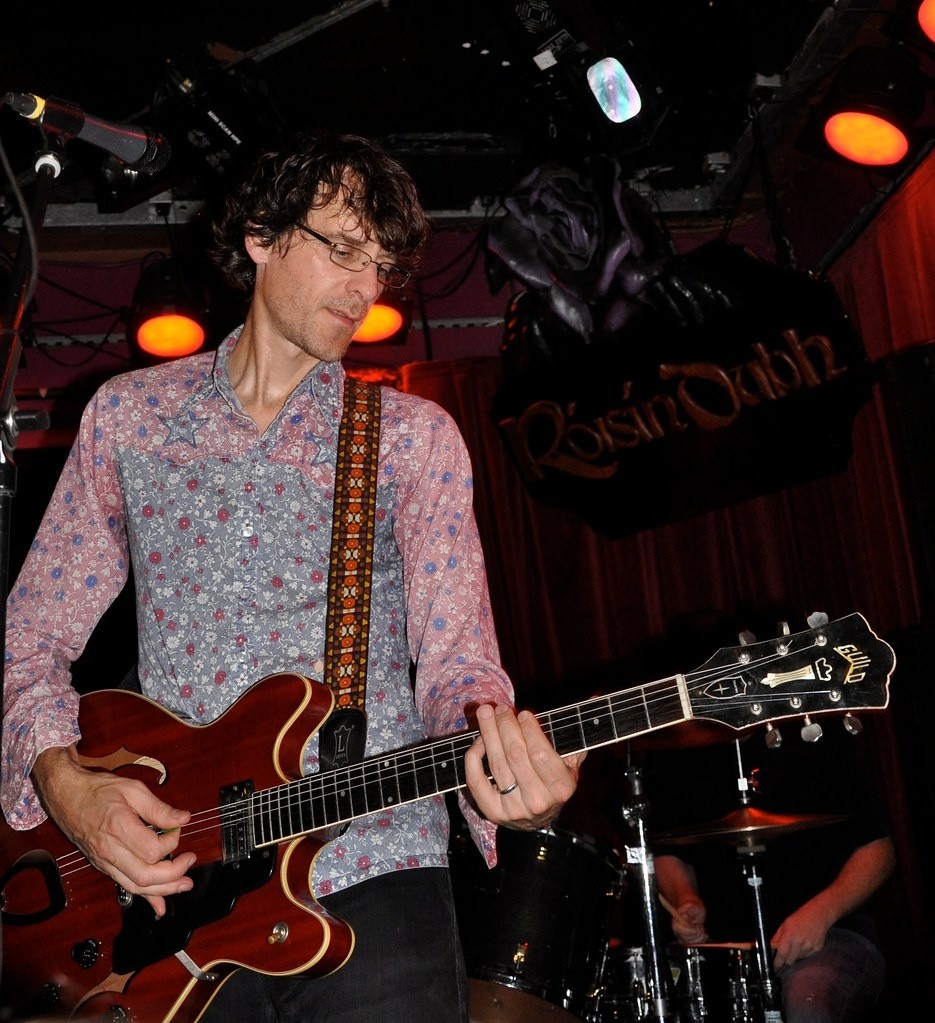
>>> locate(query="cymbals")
[646,804,855,846]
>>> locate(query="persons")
[634,612,895,1023]
[1,133,588,1022]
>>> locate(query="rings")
[500,781,518,794]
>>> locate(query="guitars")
[0,607,896,1023]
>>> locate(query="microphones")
[3,92,172,175]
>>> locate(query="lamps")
[134,263,208,355]
[356,287,405,344]
[821,44,923,166]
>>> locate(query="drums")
[593,943,764,1023]
[447,813,627,1023]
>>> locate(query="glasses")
[295,223,413,289]
